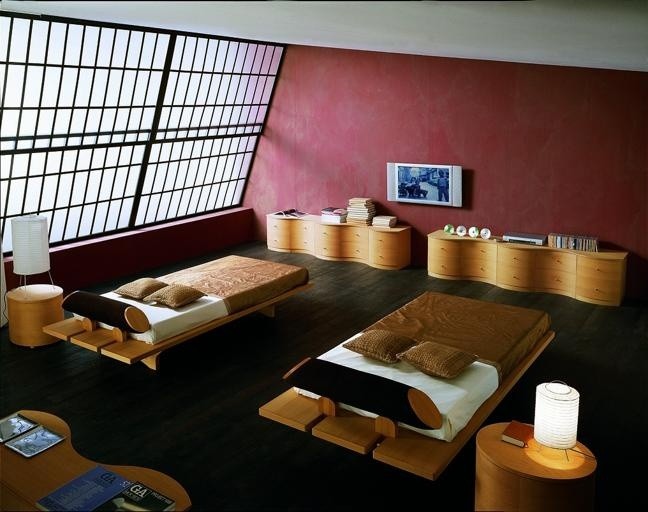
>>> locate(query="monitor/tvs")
[386,162,463,207]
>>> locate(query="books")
[275,208,307,218]
[321,207,346,225]
[501,418,533,448]
[88,480,176,512]
[35,464,134,512]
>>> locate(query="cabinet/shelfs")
[575,251,625,307]
[497,237,575,302]
[368,222,411,272]
[427,228,496,289]
[266,209,316,260]
[316,213,368,267]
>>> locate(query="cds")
[480,228,491,239]
[456,225,466,236]
[468,227,479,238]
[444,224,455,235]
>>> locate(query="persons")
[436,170,449,202]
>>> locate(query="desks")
[0,410,195,511]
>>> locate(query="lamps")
[532,374,594,464]
[8,213,58,298]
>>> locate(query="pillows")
[61,285,150,334]
[280,352,445,429]
[141,282,205,312]
[393,331,480,381]
[113,274,171,304]
[341,321,420,366]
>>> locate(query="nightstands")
[474,419,598,511]
[4,282,67,350]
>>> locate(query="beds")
[257,291,558,510]
[41,252,314,373]
